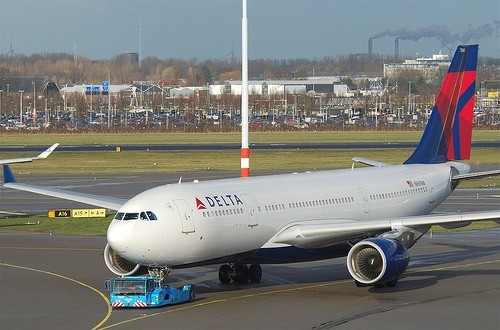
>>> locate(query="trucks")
[104,275,198,309]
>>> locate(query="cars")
[2,103,426,129]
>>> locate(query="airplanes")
[0,142,60,166]
[1,43,500,290]
